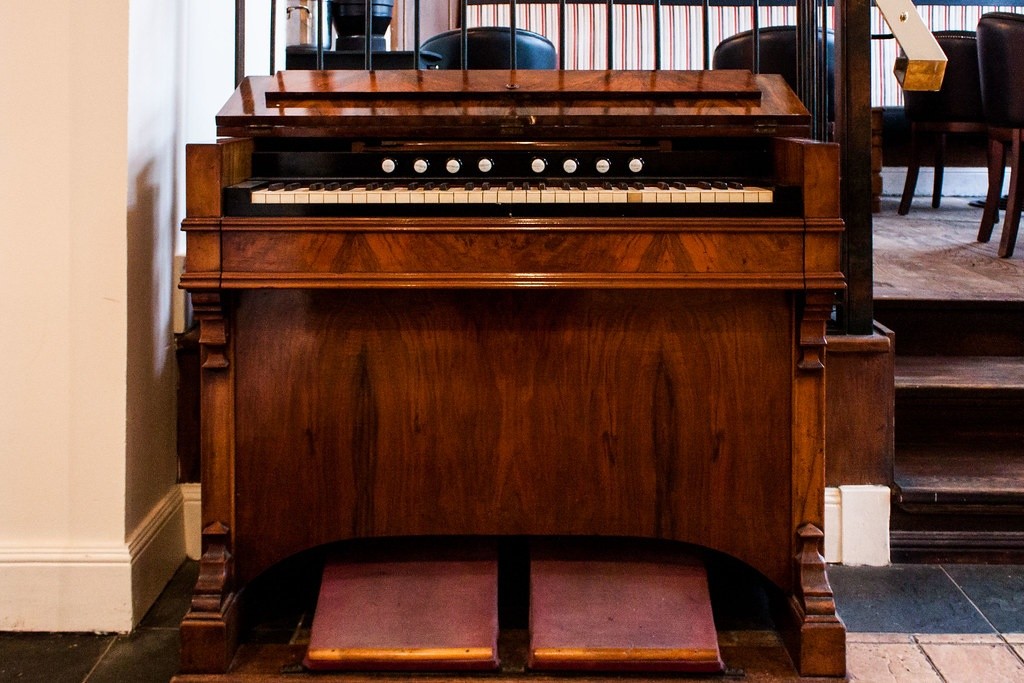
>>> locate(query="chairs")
[419,27,557,70]
[898,12,1024,258]
[713,26,835,124]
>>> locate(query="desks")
[287,49,443,71]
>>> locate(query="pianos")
[167,65,848,681]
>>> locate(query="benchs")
[871,106,1013,214]
